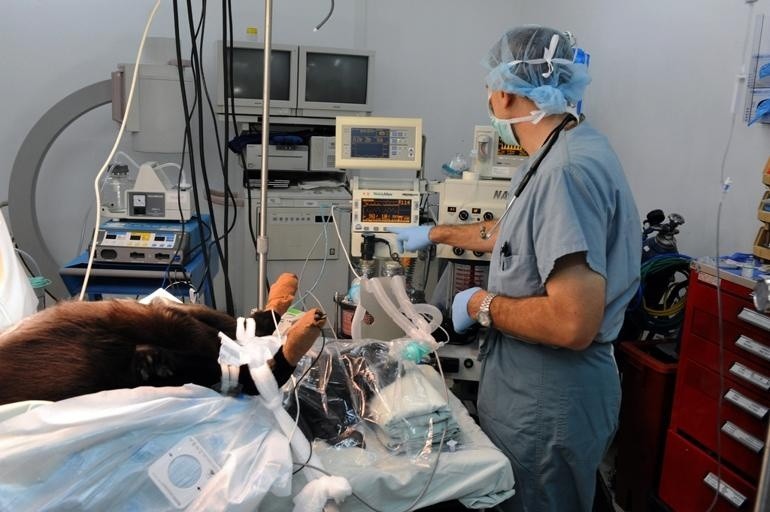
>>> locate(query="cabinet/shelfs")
[657,257,769,512]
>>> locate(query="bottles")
[101,163,132,213]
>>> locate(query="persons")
[386,25,643,512]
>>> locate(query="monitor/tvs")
[297,45,377,111]
[216,39,299,108]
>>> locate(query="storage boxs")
[621,340,677,432]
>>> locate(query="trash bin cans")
[614,337,681,512]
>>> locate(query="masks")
[486,67,520,149]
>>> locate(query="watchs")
[476,293,500,330]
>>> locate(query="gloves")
[450,286,482,336]
[383,226,434,254]
